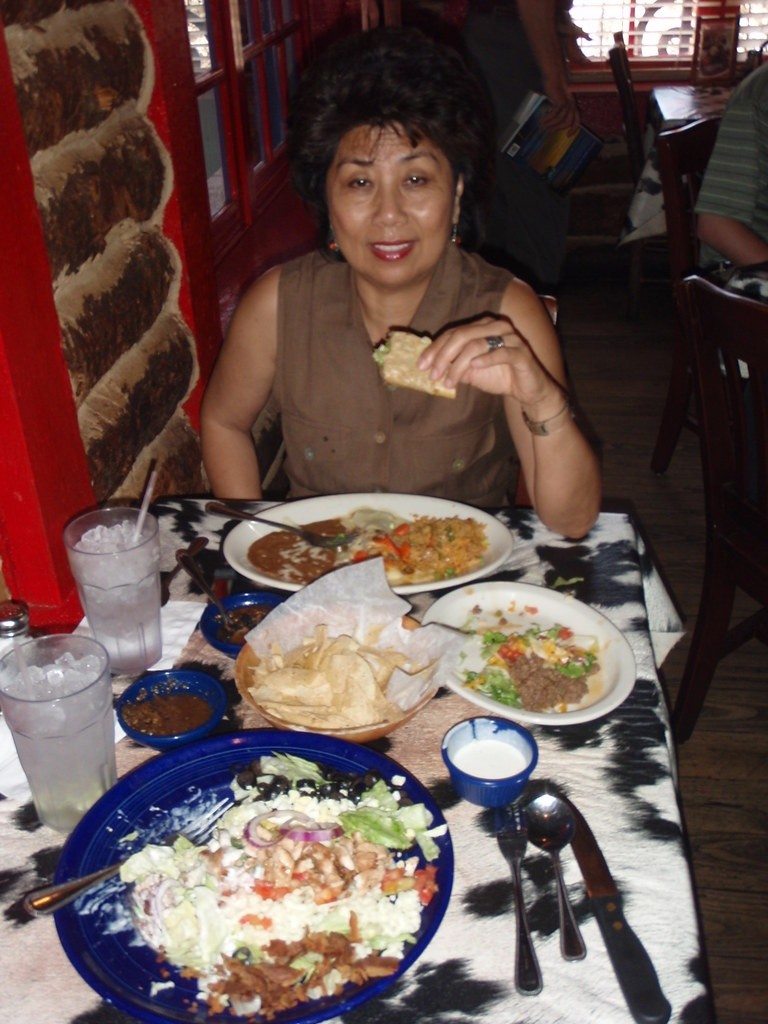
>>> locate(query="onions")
[244,808,314,848]
[275,820,344,842]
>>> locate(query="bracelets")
[522,401,573,435]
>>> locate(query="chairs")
[671,273,768,746]
[608,32,681,320]
[649,116,724,475]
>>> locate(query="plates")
[223,492,514,596]
[421,581,638,726]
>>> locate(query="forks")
[22,797,237,916]
[205,502,359,548]
[493,805,544,996]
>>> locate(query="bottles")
[0,599,36,657]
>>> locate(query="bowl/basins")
[440,716,540,807]
[51,727,454,1024]
[200,592,287,659]
[233,613,440,743]
[116,669,227,751]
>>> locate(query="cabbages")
[118,750,442,980]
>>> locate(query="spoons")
[524,794,587,961]
[175,548,245,639]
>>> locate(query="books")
[499,93,600,190]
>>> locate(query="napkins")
[0,710,127,806]
[244,557,477,710]
[72,600,207,671]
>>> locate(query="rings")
[486,336,504,350]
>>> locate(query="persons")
[460,1,595,294]
[694,62,768,284]
[201,25,601,538]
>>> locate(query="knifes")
[554,792,672,1024]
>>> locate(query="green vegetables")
[467,630,595,707]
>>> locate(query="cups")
[0,633,118,833]
[62,506,163,674]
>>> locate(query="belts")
[469,4,515,17]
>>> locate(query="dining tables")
[0,496,712,1024]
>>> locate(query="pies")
[241,624,423,730]
[379,331,456,400]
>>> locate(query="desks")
[645,85,739,147]
